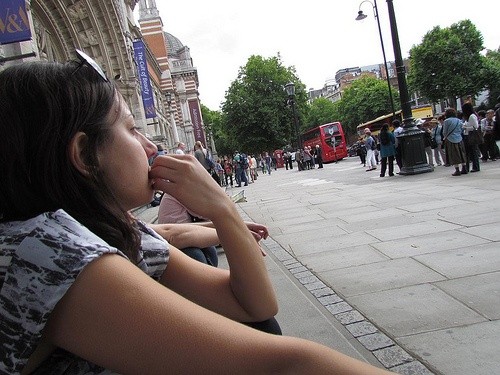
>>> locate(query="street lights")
[284,83,305,168]
[354,0,396,120]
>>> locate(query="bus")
[356,105,434,140]
[298,121,348,164]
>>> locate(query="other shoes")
[396,171,400,174]
[361,162,378,171]
[429,163,446,166]
[461,169,468,174]
[389,173,394,176]
[452,170,461,176]
[470,168,480,172]
[468,155,500,163]
[380,174,385,177]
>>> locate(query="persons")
[259,148,293,175]
[0,49,402,375]
[158,192,222,249]
[416,101,500,176]
[149,145,165,165]
[346,120,404,177]
[188,141,259,187]
[176,143,186,154]
[121,211,269,268]
[295,145,323,171]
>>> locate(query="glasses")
[69,47,111,86]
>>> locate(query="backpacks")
[235,155,249,169]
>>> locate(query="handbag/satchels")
[376,143,381,151]
[429,140,438,149]
[368,141,377,150]
[468,125,484,145]
